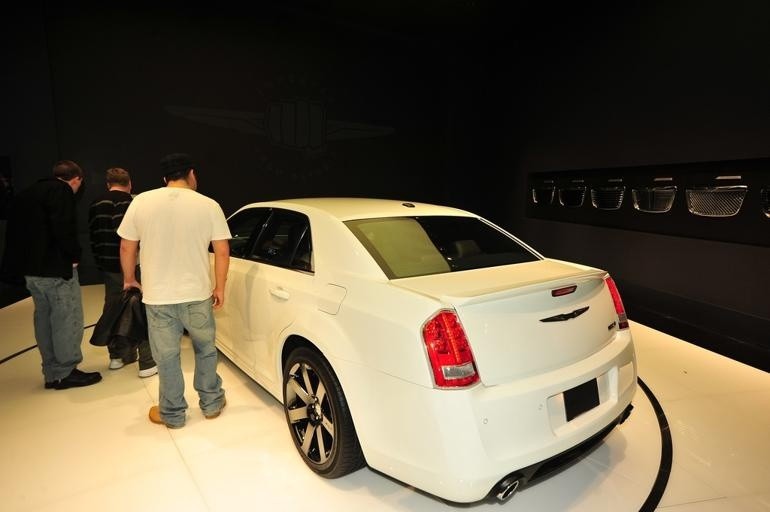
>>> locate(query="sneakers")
[109,352,159,378]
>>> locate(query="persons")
[116,153,233,429]
[87,167,158,379]
[1,160,101,389]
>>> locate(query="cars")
[207,195,637,506]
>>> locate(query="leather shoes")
[44,359,102,390]
[203,395,227,419]
[149,406,176,428]
